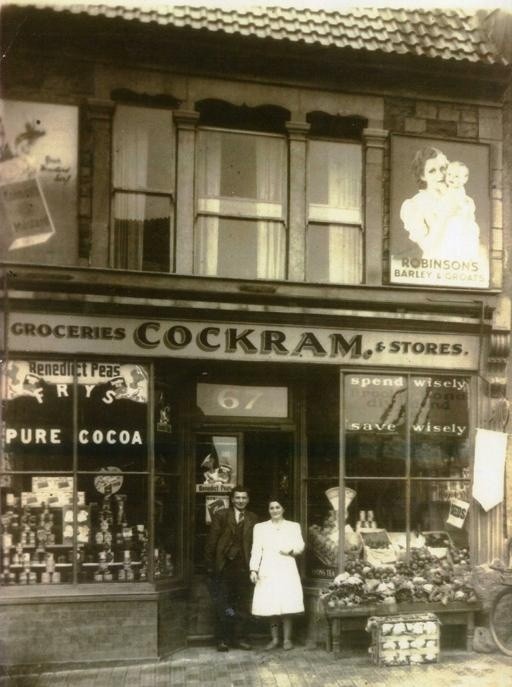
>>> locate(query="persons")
[247,495,307,650]
[399,144,481,286]
[434,161,470,214]
[201,485,261,650]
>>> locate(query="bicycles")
[487,562,512,657]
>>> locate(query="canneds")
[0,542,135,584]
[355,510,377,530]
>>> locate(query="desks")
[326,600,482,658]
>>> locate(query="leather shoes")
[216,641,229,651]
[284,641,292,650]
[267,640,281,650]
[233,641,252,650]
